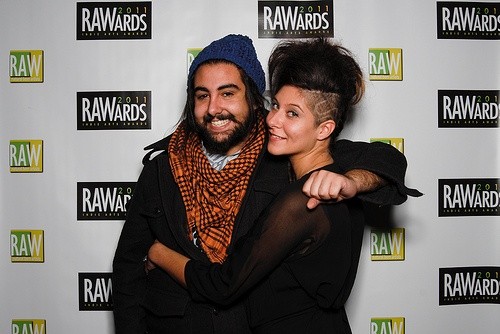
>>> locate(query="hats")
[188,34,266,96]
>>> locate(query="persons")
[113,34,407,334]
[127,35,366,334]
